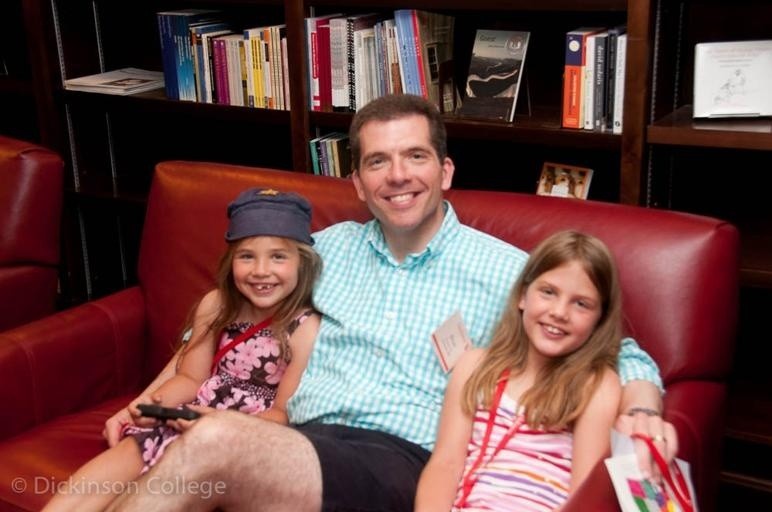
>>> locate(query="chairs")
[0,137,66,335]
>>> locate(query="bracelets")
[626,406,659,416]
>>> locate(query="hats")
[224,188,315,247]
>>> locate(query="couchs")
[0,160,740,512]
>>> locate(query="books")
[309,131,353,179]
[62,9,626,136]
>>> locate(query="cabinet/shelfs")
[24,0,772,493]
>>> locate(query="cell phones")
[137,403,198,420]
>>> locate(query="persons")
[412,228,624,509]
[102,92,682,510]
[36,185,325,512]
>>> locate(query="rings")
[650,436,667,443]
